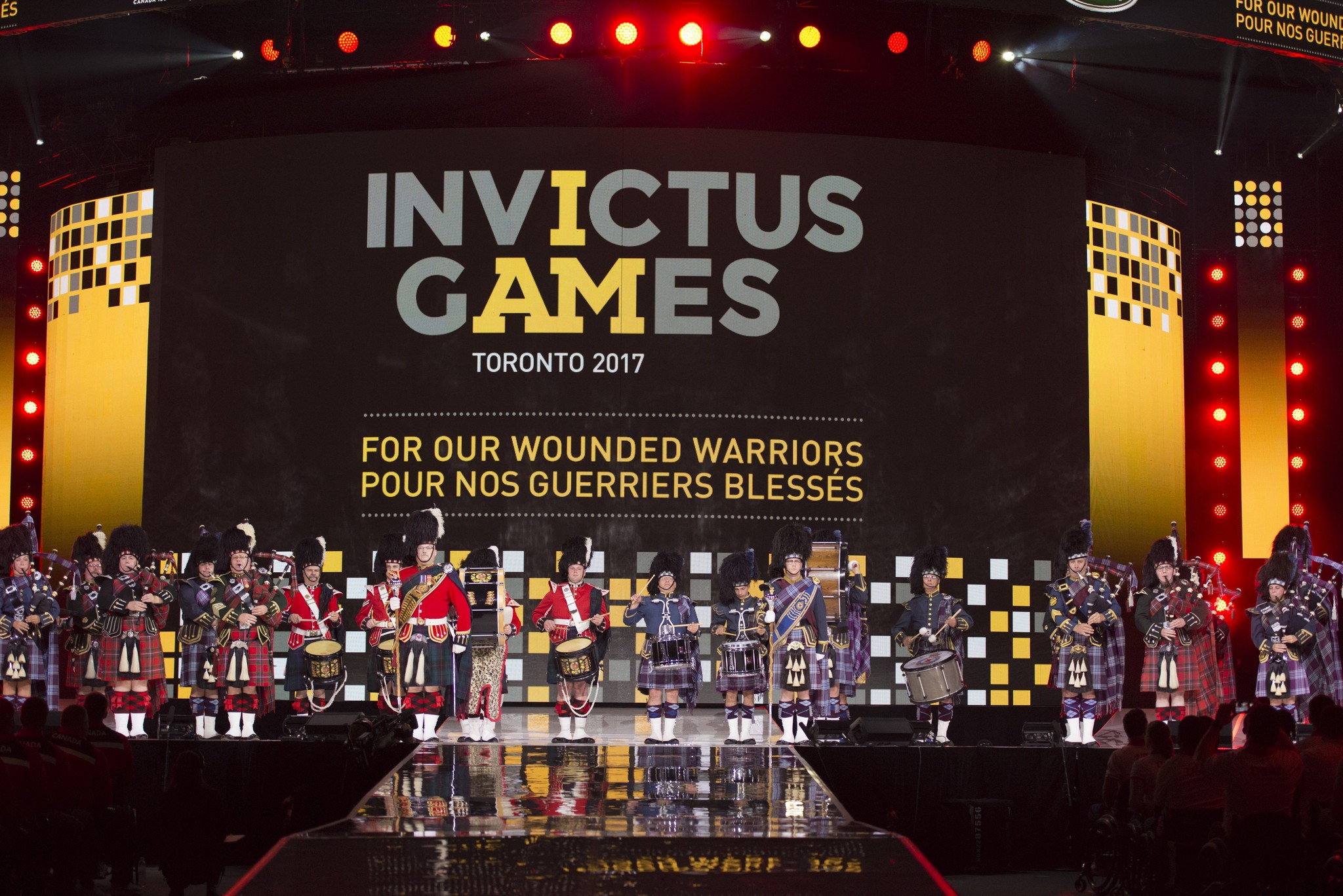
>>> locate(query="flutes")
[1274,520,1343,663]
[96,523,103,576]
[17,510,79,646]
[244,518,296,608]
[1078,518,1134,626]
[126,549,174,626]
[199,523,219,579]
[1164,520,1242,644]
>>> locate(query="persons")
[1043,518,1343,895]
[397,744,840,839]
[0,507,522,896]
[710,524,871,745]
[532,536,612,743]
[623,550,703,744]
[892,545,974,747]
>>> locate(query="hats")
[1142,537,1183,586]
[646,523,849,607]
[1254,525,1309,602]
[1061,520,1093,561]
[70,518,255,576]
[289,536,326,586]
[558,536,593,578]
[373,508,499,585]
[1181,564,1201,590]
[0,524,32,563]
[909,545,948,596]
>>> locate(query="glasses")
[785,559,801,565]
[1156,565,1173,572]
[922,575,938,580]
[87,564,103,569]
[232,555,249,560]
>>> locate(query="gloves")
[848,560,860,574]
[390,597,400,611]
[452,644,467,654]
[765,610,776,623]
[1317,606,1328,621]
[816,653,825,661]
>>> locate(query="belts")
[184,617,447,637]
[796,619,810,627]
[554,619,575,627]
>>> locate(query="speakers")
[14,710,63,728]
[848,717,914,745]
[305,712,366,737]
[157,714,196,739]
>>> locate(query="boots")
[106,711,1223,748]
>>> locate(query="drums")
[458,566,507,648]
[899,648,967,706]
[376,638,396,678]
[303,638,345,686]
[554,636,600,683]
[720,640,764,678]
[650,633,694,672]
[802,541,849,623]
